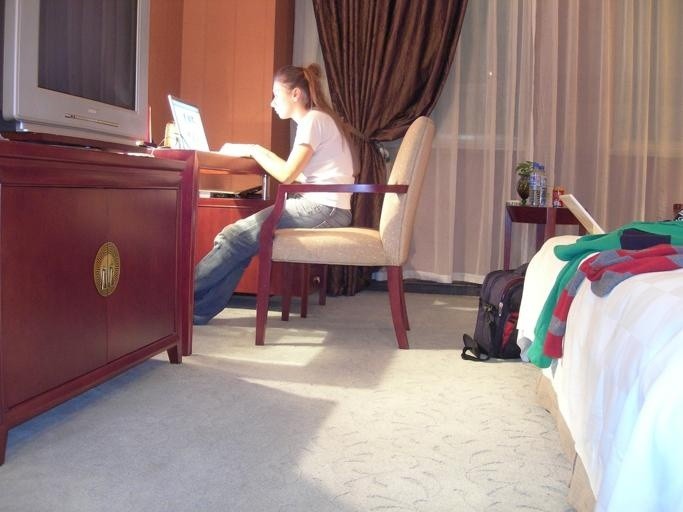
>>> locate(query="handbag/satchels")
[473,262,529,359]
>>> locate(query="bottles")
[539,166,547,207]
[529,163,540,207]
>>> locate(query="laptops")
[559,193,605,237]
[168,94,215,152]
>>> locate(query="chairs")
[255,115,436,351]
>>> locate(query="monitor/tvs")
[0,0,151,147]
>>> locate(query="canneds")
[553,188,564,206]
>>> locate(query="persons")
[193,64,356,325]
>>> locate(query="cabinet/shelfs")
[0,139,199,464]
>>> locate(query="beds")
[517,223,683,512]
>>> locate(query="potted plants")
[513,161,535,202]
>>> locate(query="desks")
[150,145,308,321]
[503,205,587,269]
